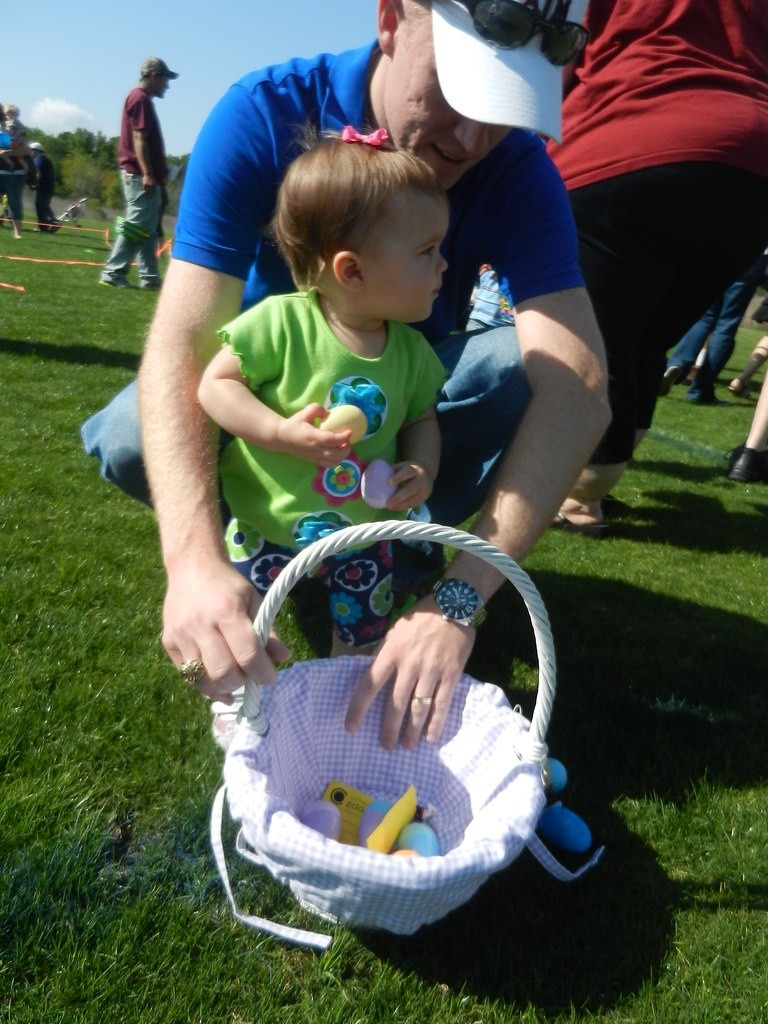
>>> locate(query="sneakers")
[141,278,163,291]
[99,272,138,291]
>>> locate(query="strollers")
[52,197,89,230]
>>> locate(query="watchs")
[430,576,487,631]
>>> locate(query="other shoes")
[663,363,679,398]
[725,442,768,481]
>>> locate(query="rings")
[412,694,435,708]
[182,659,205,681]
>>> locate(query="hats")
[428,0,588,145]
[140,56,179,78]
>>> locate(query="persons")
[78,1,618,750]
[657,248,768,404]
[536,1,768,538]
[99,54,180,287]
[466,262,503,331]
[680,327,768,395]
[190,128,454,671]
[0,104,29,172]
[720,374,768,485]
[0,104,28,240]
[28,140,62,233]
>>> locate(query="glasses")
[458,0,592,71]
